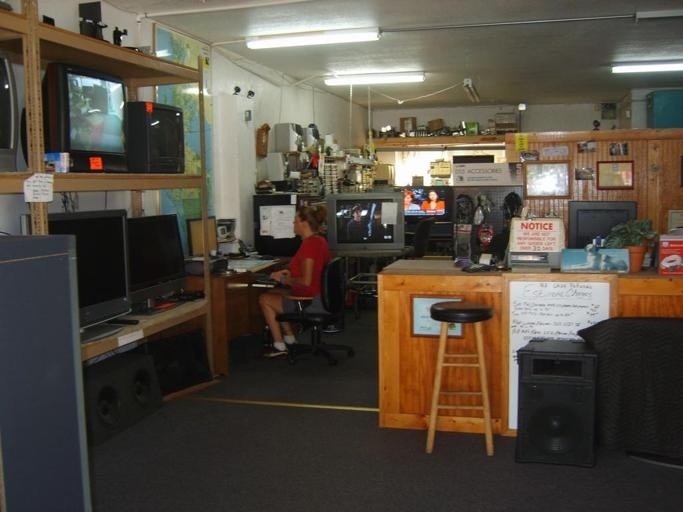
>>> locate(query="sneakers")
[264,345,288,357]
[284,342,298,348]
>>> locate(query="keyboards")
[255,274,280,286]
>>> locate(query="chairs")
[377,216,435,268]
[276,257,354,367]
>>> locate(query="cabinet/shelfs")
[35,20,217,441]
[366,135,507,148]
[0,0,42,239]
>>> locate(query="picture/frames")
[596,160,635,190]
[522,160,574,200]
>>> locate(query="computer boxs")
[187,257,229,276]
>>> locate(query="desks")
[187,258,281,375]
[377,260,683,436]
[337,246,413,319]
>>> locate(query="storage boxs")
[646,88,683,128]
[619,87,683,129]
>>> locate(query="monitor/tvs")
[124,214,188,317]
[0,56,21,173]
[186,216,219,258]
[128,100,185,174]
[19,208,133,345]
[42,62,129,172]
[400,186,454,224]
[326,191,405,250]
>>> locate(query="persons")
[258,204,330,358]
[422,191,445,214]
[367,211,385,243]
[400,189,420,217]
[344,203,368,244]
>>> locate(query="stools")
[425,302,495,456]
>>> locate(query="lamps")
[634,10,682,23]
[463,78,482,104]
[609,59,683,75]
[324,71,430,87]
[245,27,382,50]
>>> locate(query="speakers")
[84,352,165,449]
[512,340,600,470]
